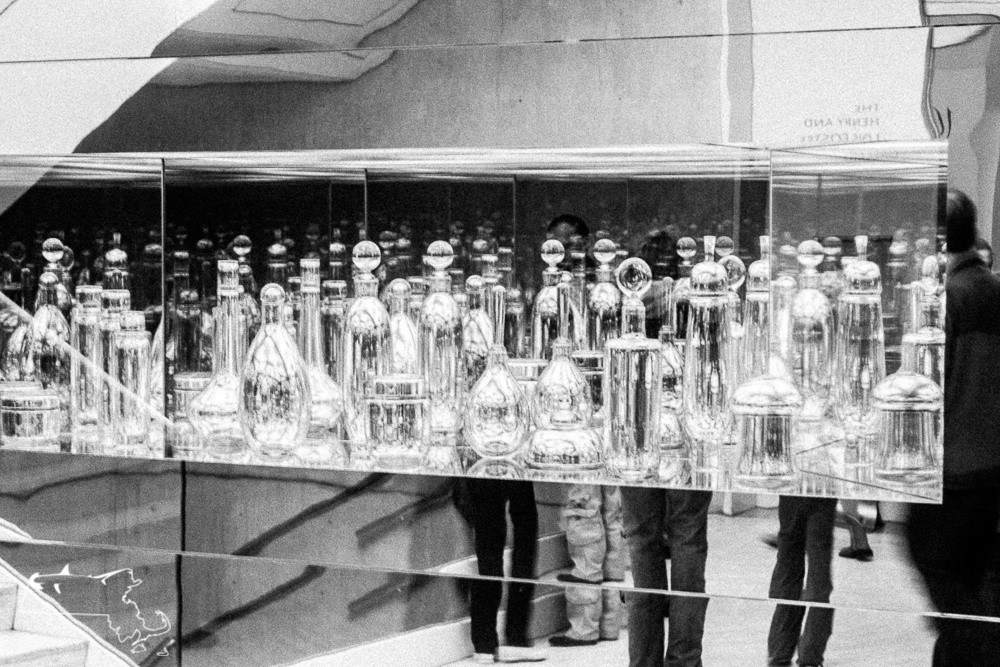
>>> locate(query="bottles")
[1,218,947,504]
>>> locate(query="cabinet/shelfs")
[0,0,1000,667]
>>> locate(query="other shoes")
[839,546,873,562]
[759,533,778,549]
[495,641,549,662]
[547,634,601,647]
[472,652,495,665]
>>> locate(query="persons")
[765,604,835,667]
[447,477,539,578]
[464,580,547,663]
[548,584,621,647]
[898,183,999,617]
[624,591,710,667]
[769,495,838,603]
[619,486,709,592]
[762,499,875,559]
[556,481,626,584]
